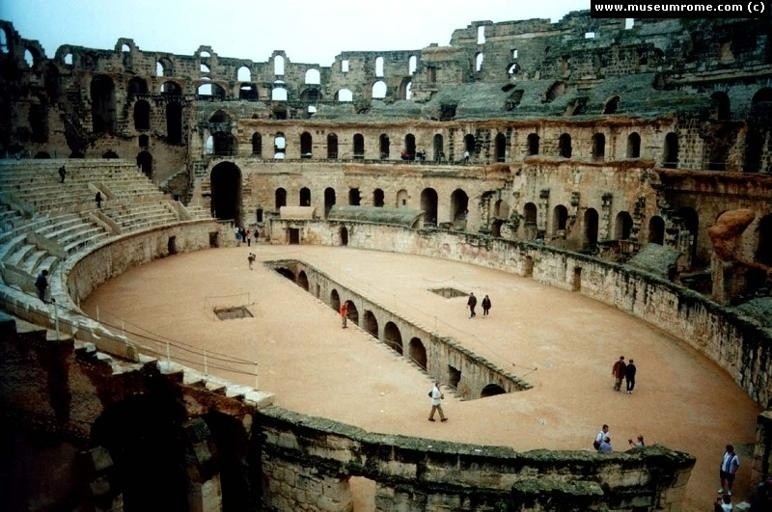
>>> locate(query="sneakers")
[717,488,732,496]
[440,417,449,422]
[428,418,436,422]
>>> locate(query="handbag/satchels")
[593,440,601,450]
[428,390,433,398]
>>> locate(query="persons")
[95,191,104,207]
[593,424,610,451]
[239,227,246,243]
[340,302,349,330]
[480,294,491,315]
[612,355,626,392]
[628,434,644,448]
[246,229,251,248]
[234,225,242,240]
[427,382,448,422]
[248,252,256,270]
[467,292,477,317]
[713,494,732,511]
[624,359,636,394]
[599,437,612,453]
[34,270,50,304]
[58,165,67,184]
[717,443,739,494]
[253,230,259,242]
